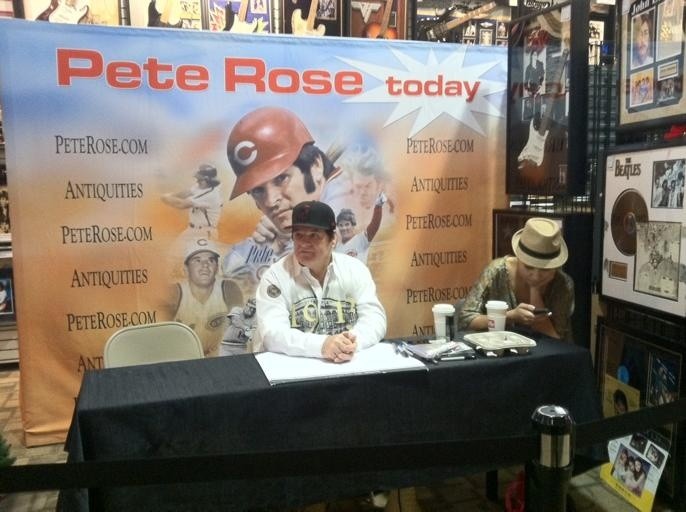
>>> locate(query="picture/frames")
[14,0,126,27]
[345,1,408,39]
[601,138,685,325]
[613,0,685,131]
[279,0,345,37]
[507,2,587,194]
[0,268,16,319]
[124,0,205,31]
[592,315,685,491]
[408,2,509,45]
[204,0,279,34]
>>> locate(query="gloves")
[376,192,387,207]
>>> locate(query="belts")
[188,223,202,229]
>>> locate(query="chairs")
[104,323,205,367]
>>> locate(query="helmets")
[227,105,315,201]
[335,211,356,226]
[246,297,257,309]
[197,164,218,177]
[183,237,221,264]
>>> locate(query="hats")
[511,216,568,269]
[283,201,336,230]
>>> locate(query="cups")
[484,300,509,332]
[432,303,455,342]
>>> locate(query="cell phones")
[534,308,548,315]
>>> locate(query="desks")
[55,328,592,511]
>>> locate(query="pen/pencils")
[440,355,476,361]
[397,340,424,362]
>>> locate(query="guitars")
[518,48,569,186]
[224,1,259,33]
[148,1,186,27]
[287,0,327,36]
[362,0,398,39]
[35,0,89,25]
[416,0,497,41]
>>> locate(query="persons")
[223,106,352,279]
[611,448,646,497]
[457,217,575,346]
[255,201,386,363]
[613,389,628,416]
[337,192,388,263]
[647,447,663,468]
[0,189,11,234]
[632,13,654,107]
[653,159,686,208]
[160,162,263,357]
[522,48,545,97]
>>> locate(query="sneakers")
[366,490,389,508]
